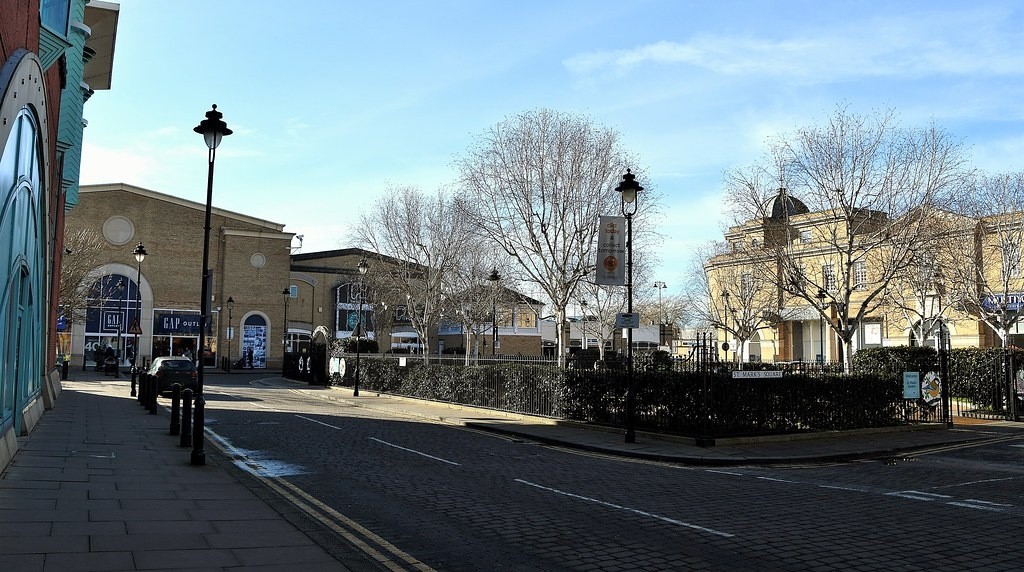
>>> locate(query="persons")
[94,347,104,371]
[105,347,115,357]
[246,349,254,369]
[185,348,190,356]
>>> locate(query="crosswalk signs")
[128,318,142,334]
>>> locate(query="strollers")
[233,357,244,369]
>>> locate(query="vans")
[391,332,428,354]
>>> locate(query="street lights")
[131,241,149,396]
[227,295,234,372]
[486,267,503,355]
[353,259,370,396]
[653,281,667,346]
[615,167,644,443]
[191,103,234,466]
[282,285,291,361]
[721,288,730,362]
[115,277,126,378]
[815,290,827,364]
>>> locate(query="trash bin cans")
[283,352,311,381]
[55,360,70,380]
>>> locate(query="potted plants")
[55,356,70,381]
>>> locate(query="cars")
[147,356,198,393]
